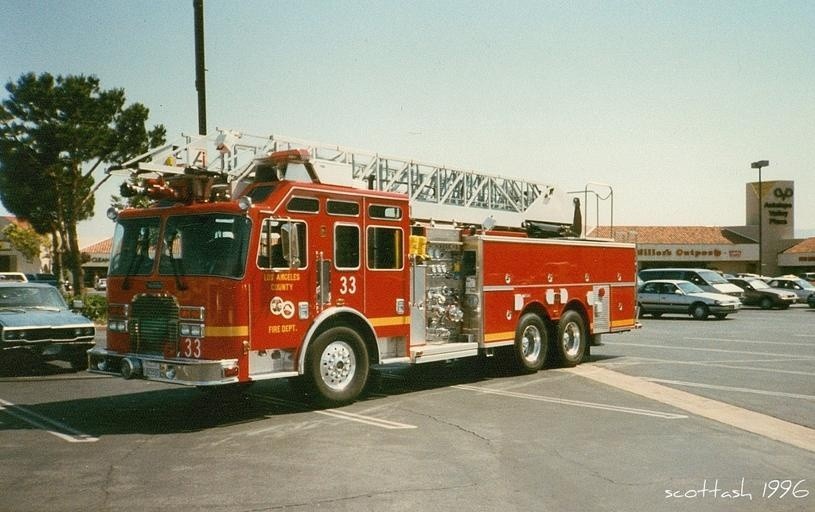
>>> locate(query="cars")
[636,280,742,321]
[723,272,815,310]
[1,272,95,373]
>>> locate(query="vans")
[637,268,744,301]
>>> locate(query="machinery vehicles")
[89,127,639,407]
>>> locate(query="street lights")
[751,161,769,278]
